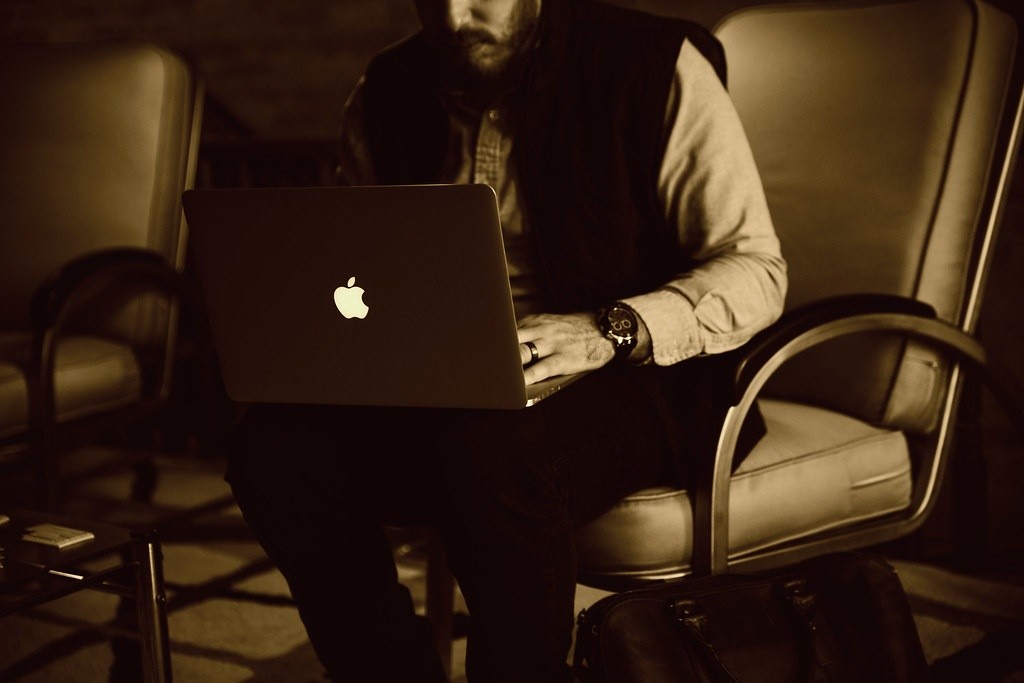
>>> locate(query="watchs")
[591,303,639,369]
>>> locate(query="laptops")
[183,184,593,411]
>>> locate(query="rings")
[525,342,538,367]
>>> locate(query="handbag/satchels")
[571,551,930,683]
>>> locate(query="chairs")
[0,45,204,683]
[396,0,1024,683]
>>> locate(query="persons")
[229,1,788,682]
